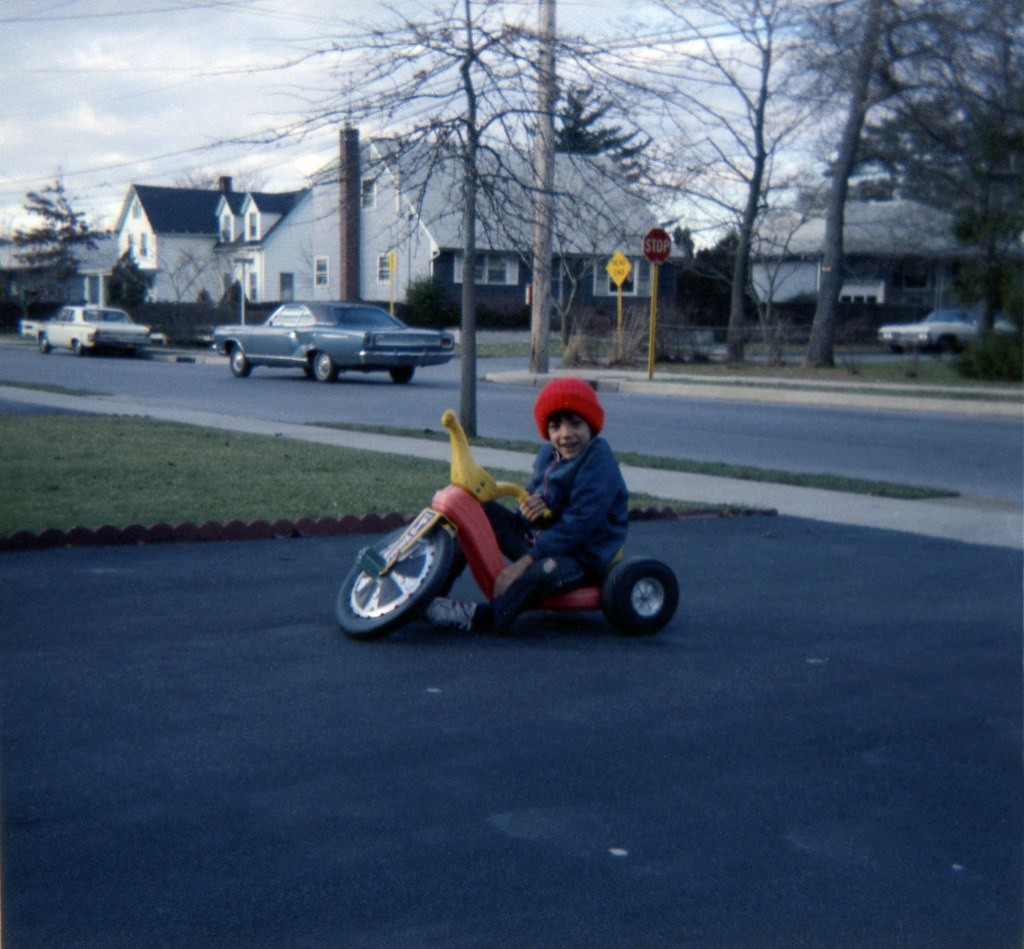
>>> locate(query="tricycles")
[334,408,680,643]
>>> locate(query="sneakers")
[426,597,477,631]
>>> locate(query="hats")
[533,377,605,441]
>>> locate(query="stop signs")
[644,227,673,261]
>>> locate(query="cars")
[212,298,457,385]
[878,311,1015,357]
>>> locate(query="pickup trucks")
[20,305,155,360]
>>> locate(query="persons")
[424,377,630,633]
